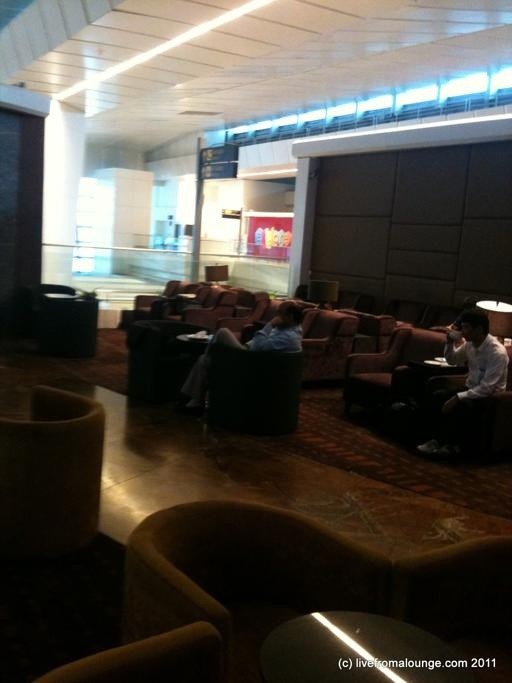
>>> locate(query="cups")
[449,329,463,340]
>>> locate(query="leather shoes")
[174,402,203,415]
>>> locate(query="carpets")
[0,326,512,521]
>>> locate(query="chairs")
[119,279,512,465]
[0,386,512,683]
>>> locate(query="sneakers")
[416,438,439,454]
[438,443,460,455]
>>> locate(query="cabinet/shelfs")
[19,281,97,355]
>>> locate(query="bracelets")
[445,333,454,343]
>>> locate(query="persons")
[415,310,510,455]
[445,295,481,332]
[181,300,306,410]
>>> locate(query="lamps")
[203,262,229,283]
